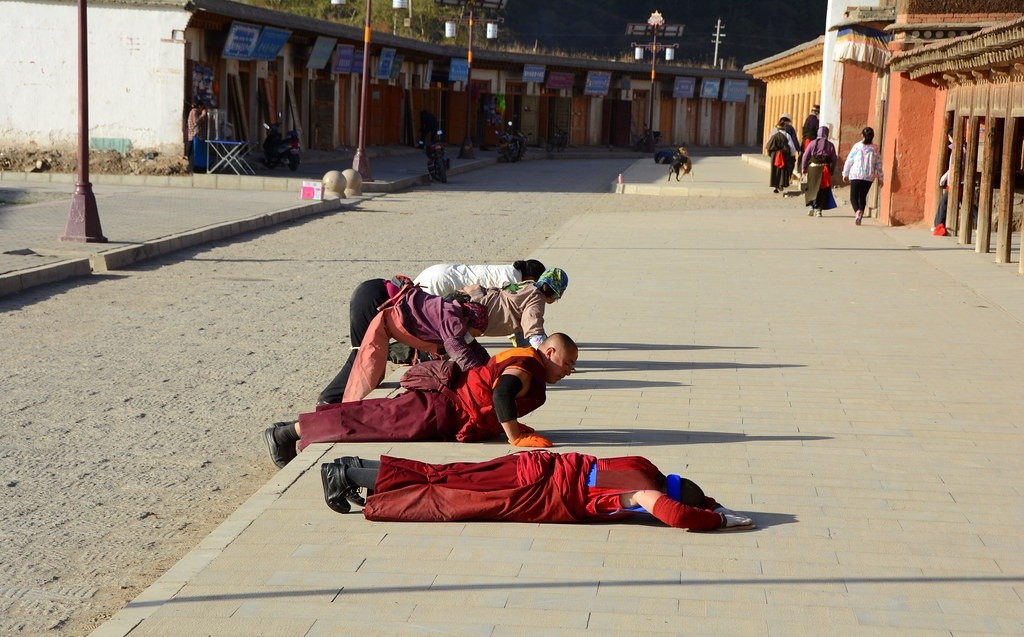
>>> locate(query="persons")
[311,257,568,405]
[184,99,206,159]
[930,128,977,232]
[261,333,579,469]
[798,126,839,218]
[797,105,822,178]
[320,449,756,532]
[841,127,885,225]
[766,113,800,194]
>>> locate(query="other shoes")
[931,225,936,233]
[854,208,864,226]
[320,463,353,514]
[814,211,823,218]
[316,396,328,406]
[333,456,365,508]
[271,422,297,461]
[262,427,290,470]
[773,185,785,193]
[807,209,815,217]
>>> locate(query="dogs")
[668,146,693,182]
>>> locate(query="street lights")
[444,1,504,159]
[630,10,679,152]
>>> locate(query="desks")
[206,140,259,176]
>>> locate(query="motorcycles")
[262,112,301,171]
[636,122,660,153]
[418,131,450,184]
[547,124,568,153]
[493,121,532,161]
